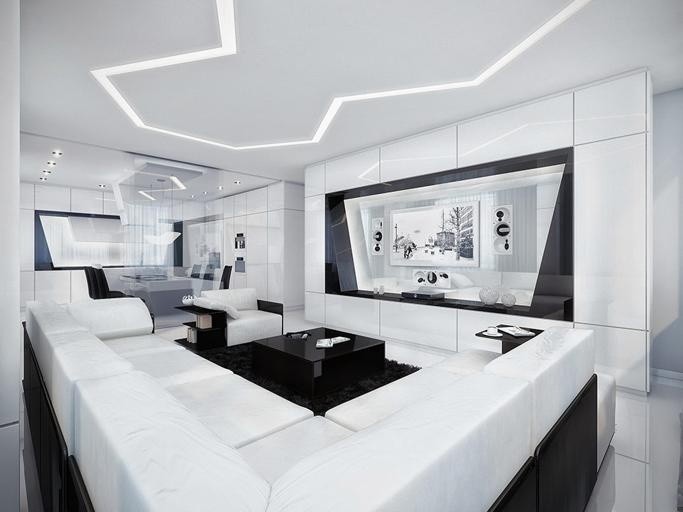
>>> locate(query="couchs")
[30,290,619,512]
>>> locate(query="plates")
[482,332,503,337]
[498,325,538,338]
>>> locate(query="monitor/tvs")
[389,201,480,267]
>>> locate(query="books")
[316,336,351,348]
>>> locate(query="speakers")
[413,270,451,289]
[371,218,384,255]
[491,205,513,255]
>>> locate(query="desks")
[120,273,195,315]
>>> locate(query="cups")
[487,326,497,335]
[374,286,384,296]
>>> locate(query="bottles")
[187,328,197,344]
[479,289,516,307]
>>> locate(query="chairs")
[82,267,123,299]
[186,263,233,291]
